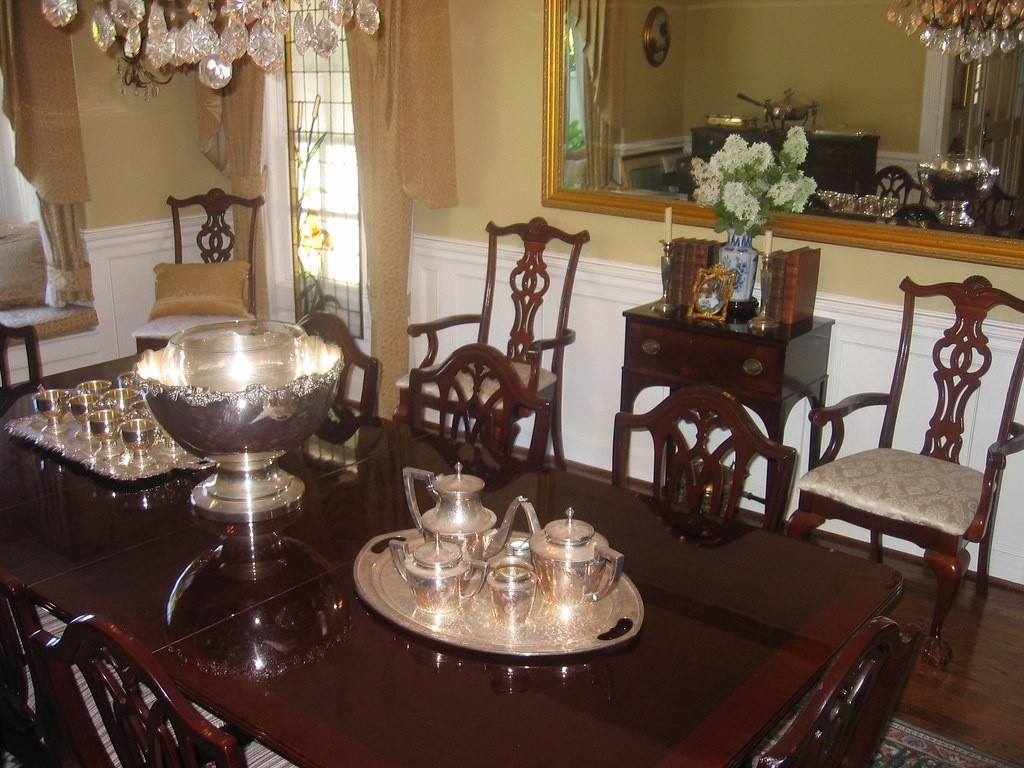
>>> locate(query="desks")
[0,353,904,768]
[620,298,836,515]
[897,203,1024,240]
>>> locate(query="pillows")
[148,261,251,321]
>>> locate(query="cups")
[105,387,141,417]
[118,371,134,388]
[76,379,112,395]
[816,190,900,218]
[129,400,147,411]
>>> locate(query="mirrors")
[542,0,1024,271]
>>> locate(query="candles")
[665,206,673,241]
[764,230,773,253]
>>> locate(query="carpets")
[0,599,1024,768]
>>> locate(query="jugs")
[518,499,624,605]
[389,531,490,614]
[402,462,528,562]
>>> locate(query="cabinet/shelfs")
[689,126,880,197]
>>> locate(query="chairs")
[784,274,1024,672]
[859,165,1020,217]
[393,217,591,472]
[755,612,922,768]
[132,187,265,355]
[0,323,40,387]
[1,570,247,768]
[296,310,381,418]
[611,383,798,532]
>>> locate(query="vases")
[718,224,760,302]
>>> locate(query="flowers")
[776,127,818,213]
[690,126,796,237]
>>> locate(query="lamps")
[885,0,1024,65]
[40,0,381,102]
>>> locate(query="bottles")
[487,560,537,626]
[505,540,531,561]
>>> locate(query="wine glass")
[88,410,125,458]
[120,420,157,468]
[134,334,344,523]
[164,507,355,696]
[69,395,100,441]
[918,153,1000,228]
[35,389,70,435]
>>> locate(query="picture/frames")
[620,145,691,194]
[686,262,736,323]
